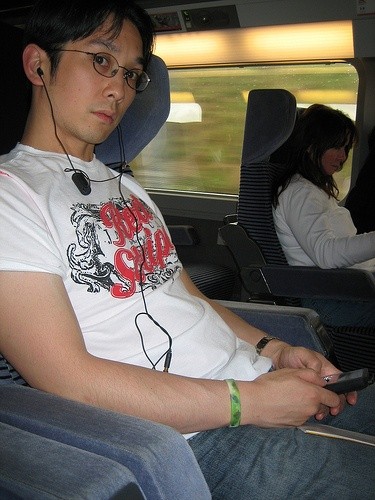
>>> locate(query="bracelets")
[226,379,241,428]
[255,337,280,355]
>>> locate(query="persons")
[0,0,375,500]
[271,104,375,277]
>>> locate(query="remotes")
[321,368,372,397]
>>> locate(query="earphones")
[37,67,44,75]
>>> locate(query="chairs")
[0,26,333,500]
[218,89,375,388]
[168,224,236,299]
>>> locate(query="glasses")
[42,47,150,92]
